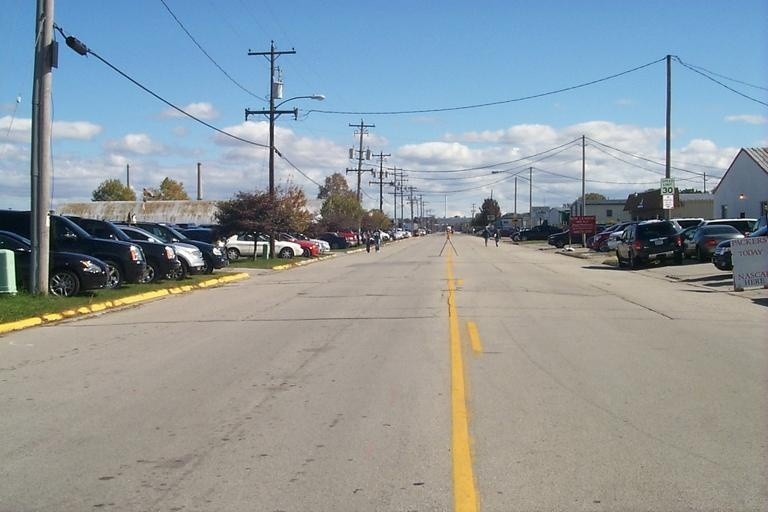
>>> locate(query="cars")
[278,232,320,257]
[110,210,430,254]
[0,229,111,297]
[212,231,305,259]
[500,218,768,271]
[115,224,206,282]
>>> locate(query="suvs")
[110,220,226,276]
[0,208,147,290]
[64,215,180,284]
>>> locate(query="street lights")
[491,171,533,213]
[269,94,326,199]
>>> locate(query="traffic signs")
[660,178,675,195]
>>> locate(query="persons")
[483,229,501,247]
[753,212,767,232]
[365,234,380,253]
